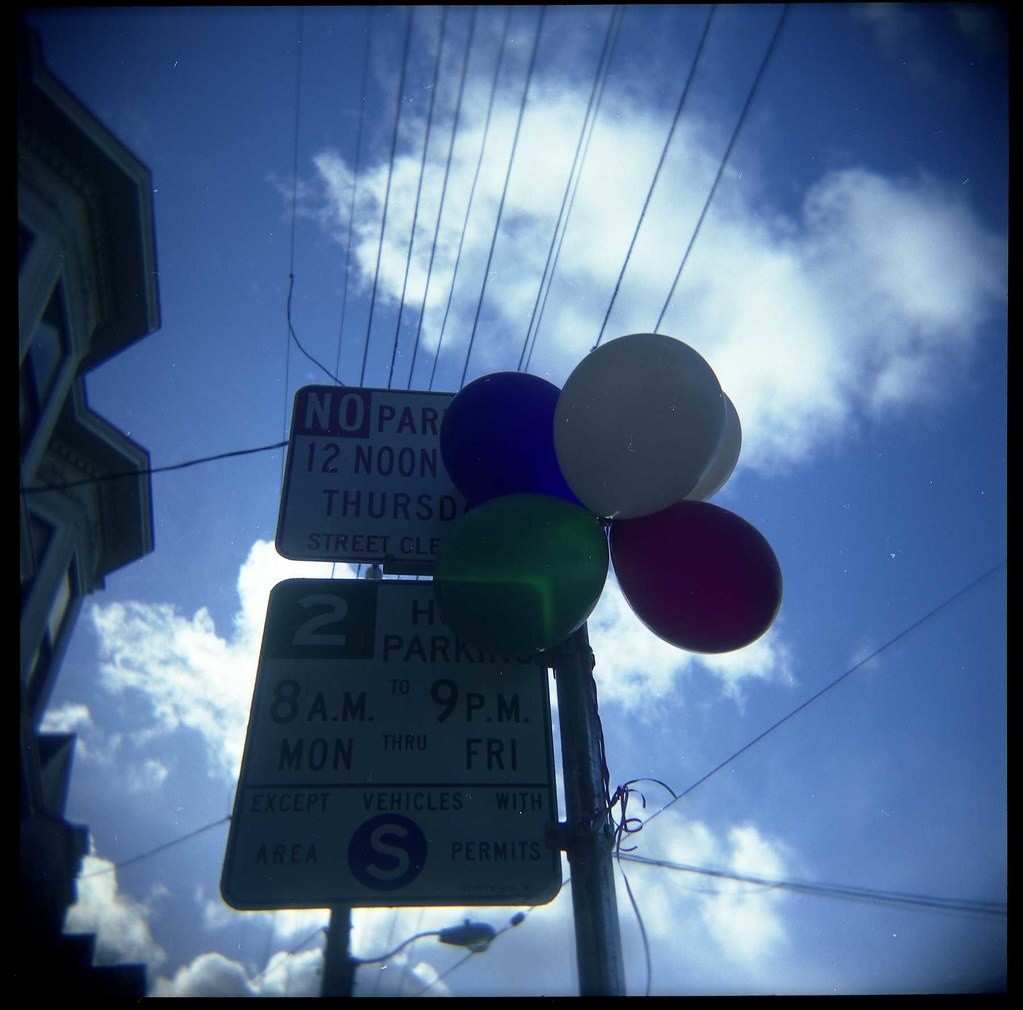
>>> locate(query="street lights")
[318,908,498,999]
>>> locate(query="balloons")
[434,494,609,658]
[438,372,587,509]
[552,334,725,522]
[684,396,743,501]
[608,500,781,654]
